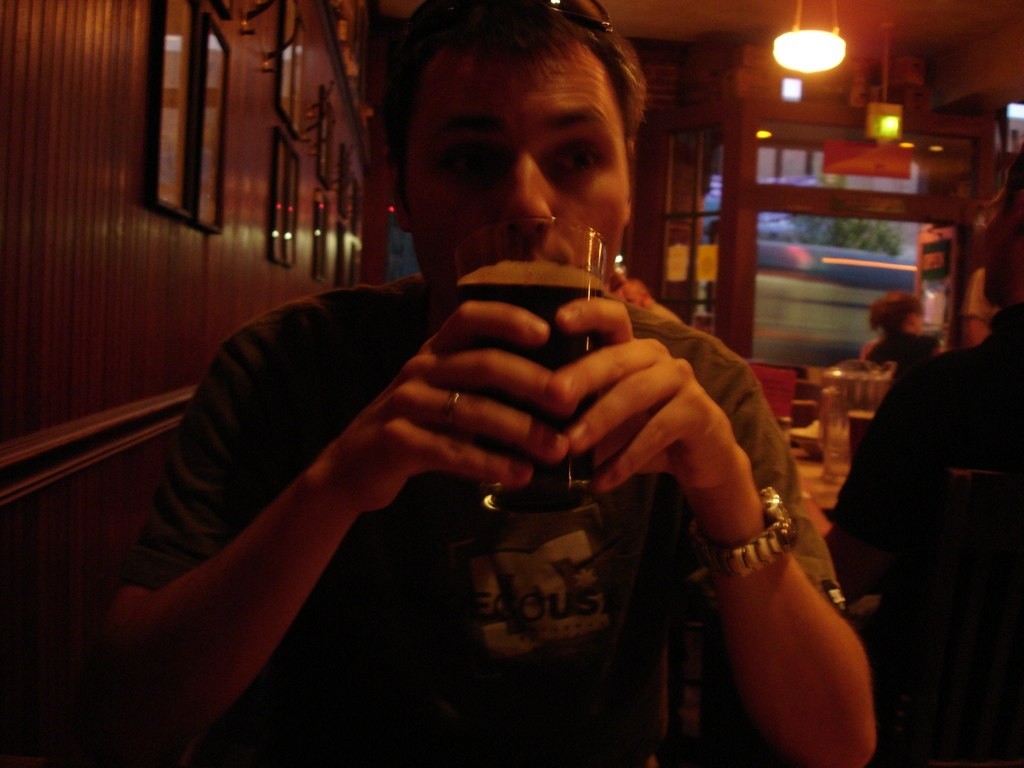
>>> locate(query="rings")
[439,389,463,426]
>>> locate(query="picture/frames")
[141,1,231,236]
[266,0,360,290]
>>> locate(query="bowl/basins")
[787,425,821,459]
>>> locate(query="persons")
[799,141,1024,768]
[105,0,880,768]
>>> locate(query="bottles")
[810,367,856,484]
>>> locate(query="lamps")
[862,30,905,144]
[772,0,848,75]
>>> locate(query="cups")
[451,214,607,517]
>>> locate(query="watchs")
[688,486,799,579]
